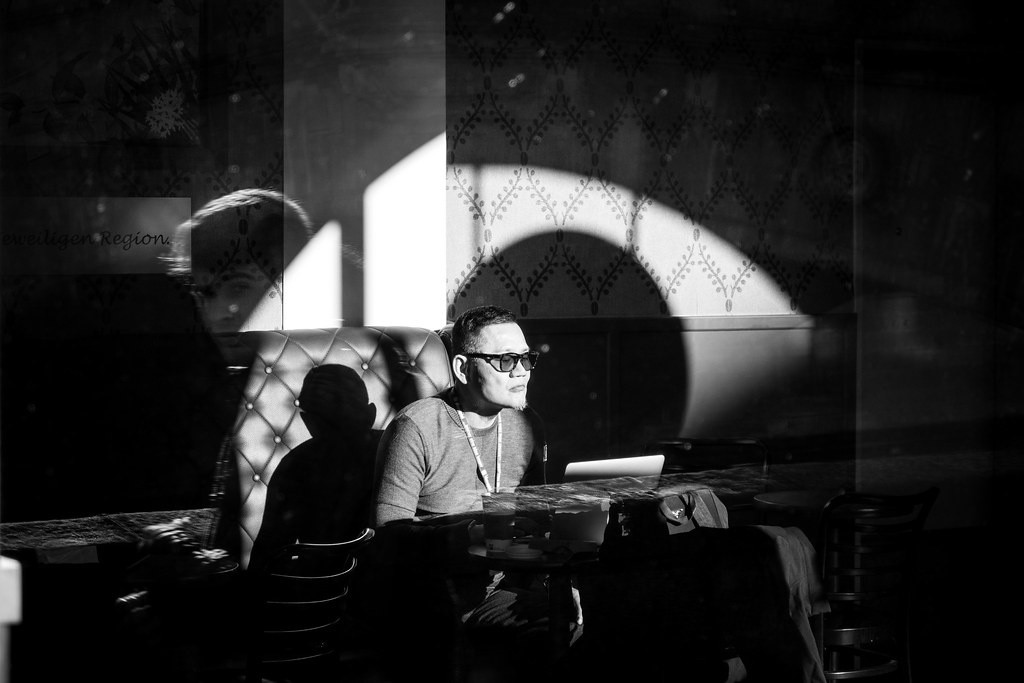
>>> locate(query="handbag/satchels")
[598,485,730,558]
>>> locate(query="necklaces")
[452,387,502,492]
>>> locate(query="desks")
[0,508,215,683]
[480,468,775,683]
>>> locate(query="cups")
[481,493,516,552]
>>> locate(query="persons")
[119,188,316,683]
[368,306,583,683]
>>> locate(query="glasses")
[463,350,540,372]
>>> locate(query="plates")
[508,549,542,558]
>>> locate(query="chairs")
[244,528,375,682]
[815,500,924,683]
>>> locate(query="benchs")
[433,317,853,471]
[217,328,455,552]
